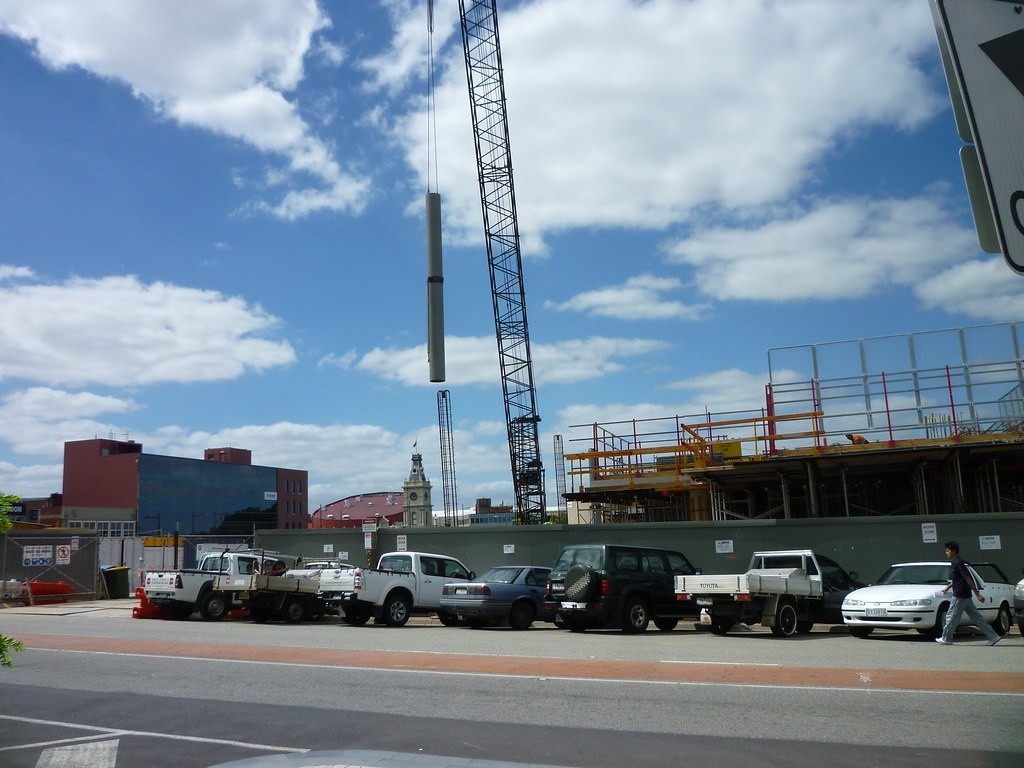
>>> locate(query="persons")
[936,541,1001,646]
[846,433,869,445]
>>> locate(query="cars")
[1013,578,1024,637]
[439,566,556,630]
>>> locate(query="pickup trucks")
[143,549,285,623]
[840,562,1015,640]
[317,552,475,625]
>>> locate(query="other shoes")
[985,636,1001,646]
[936,637,953,645]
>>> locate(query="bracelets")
[975,591,979,593]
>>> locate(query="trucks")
[673,550,875,637]
[212,559,357,623]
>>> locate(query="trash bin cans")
[105,567,132,598]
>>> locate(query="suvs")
[543,544,737,635]
